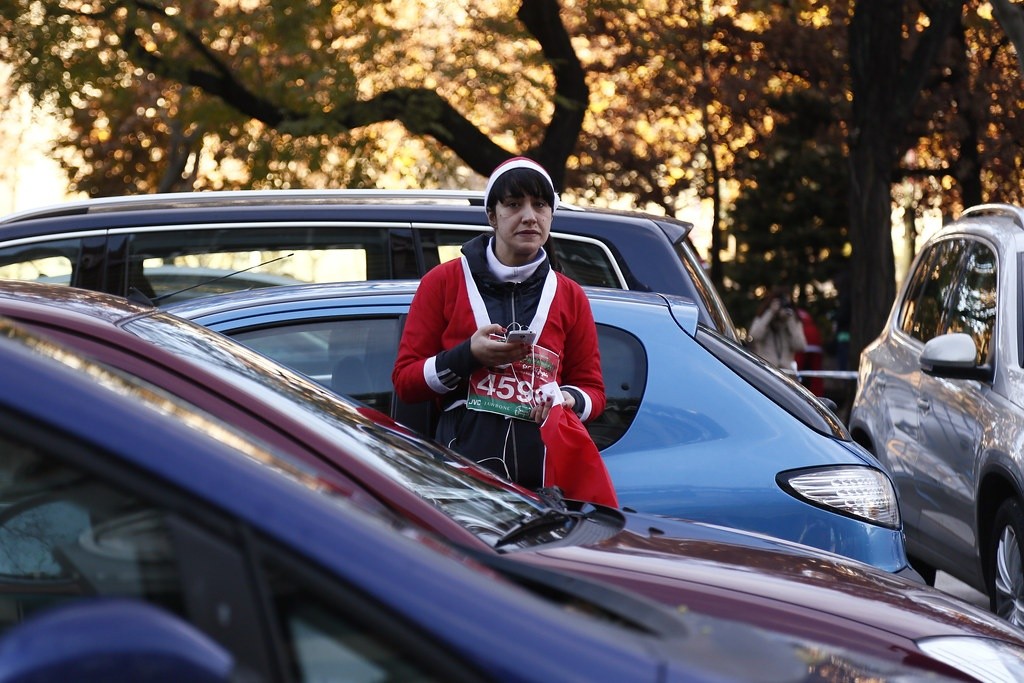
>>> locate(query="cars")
[0,278,1024,683]
[1,319,706,682]
[30,265,304,307]
[158,279,923,586]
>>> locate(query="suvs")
[0,189,748,349]
[848,202,1024,631]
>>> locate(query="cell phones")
[506,330,537,345]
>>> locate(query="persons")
[746,292,824,398]
[392,157,606,492]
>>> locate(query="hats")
[484,157,560,217]
